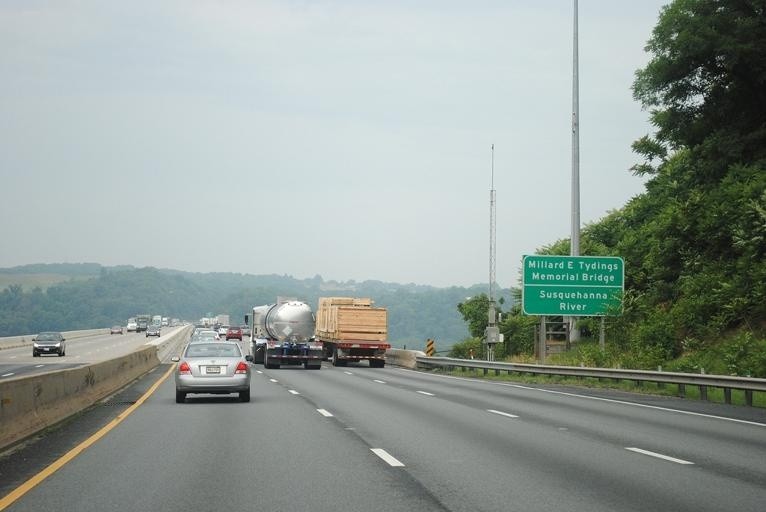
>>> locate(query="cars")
[32,331,65,356]
[172,323,253,403]
[146,325,160,337]
[111,326,123,335]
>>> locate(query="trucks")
[310,296,391,368]
[127,315,179,332]
[245,300,324,369]
[199,314,230,325]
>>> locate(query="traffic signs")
[521,255,625,318]
